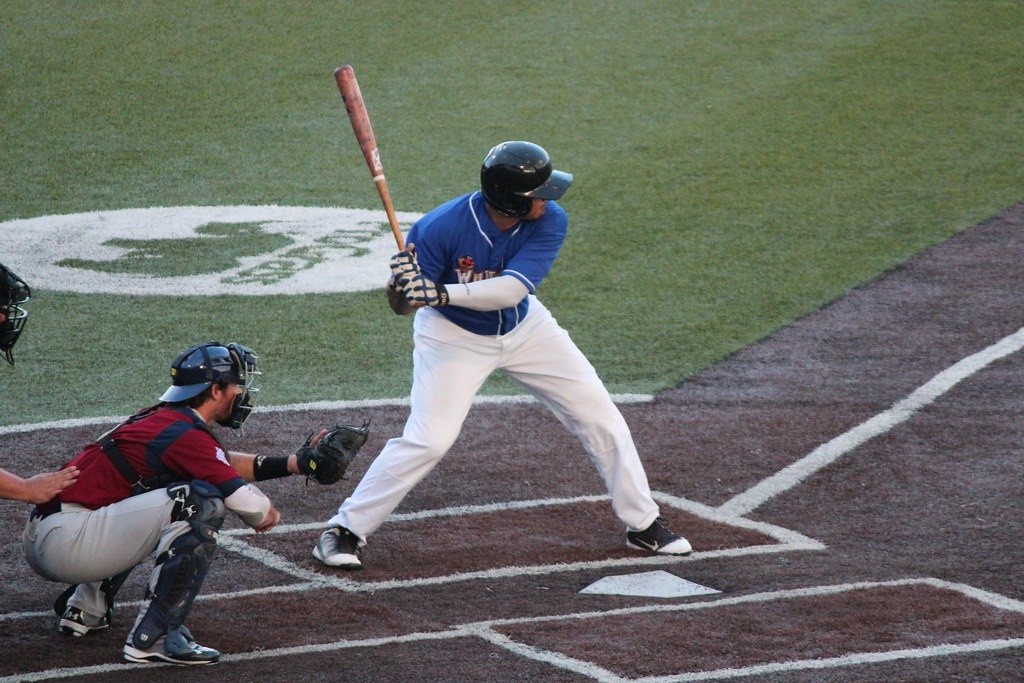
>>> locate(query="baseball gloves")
[295,423,374,486]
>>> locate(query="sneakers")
[59,605,108,636]
[311,526,362,570]
[626,518,692,556]
[121,628,221,664]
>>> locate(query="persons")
[313,142,693,570]
[0,264,79,505]
[22,341,327,666]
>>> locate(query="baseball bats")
[333,65,424,309]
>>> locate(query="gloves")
[389,243,450,309]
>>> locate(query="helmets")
[0,261,30,306]
[481,140,574,218]
[158,339,243,403]
